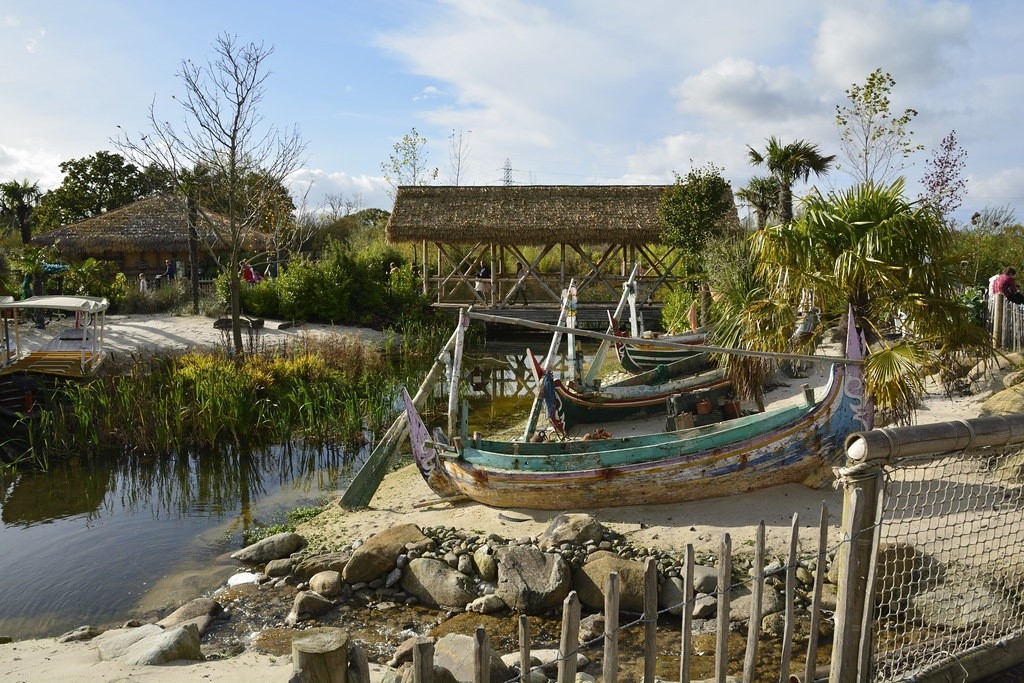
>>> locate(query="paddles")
[339,304,475,511]
[586,262,640,379]
[521,277,574,442]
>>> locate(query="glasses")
[166,262,169,263]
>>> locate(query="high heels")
[522,302,529,306]
[509,301,515,305]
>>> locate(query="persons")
[509,262,528,306]
[472,273,485,306]
[988,267,1024,305]
[410,261,419,297]
[239,258,256,283]
[264,257,277,283]
[390,262,399,275]
[479,260,489,306]
[139,273,147,293]
[156,259,178,282]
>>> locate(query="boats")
[525,286,823,432]
[400,302,877,510]
[432,380,826,471]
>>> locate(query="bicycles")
[31,308,93,327]
